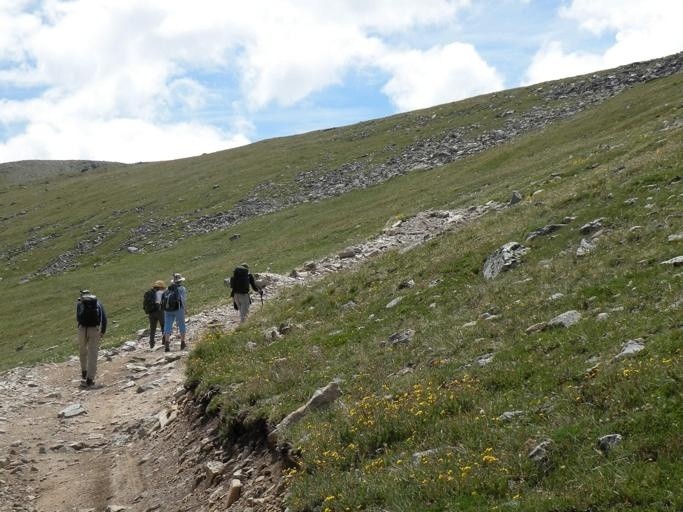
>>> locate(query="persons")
[161,273,188,353]
[231,262,263,322]
[143,279,170,347]
[76,288,107,388]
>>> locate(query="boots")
[160,335,171,352]
[179,340,187,351]
[80,369,105,390]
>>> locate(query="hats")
[151,279,167,290]
[240,263,250,269]
[169,273,186,284]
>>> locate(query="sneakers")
[149,340,155,349]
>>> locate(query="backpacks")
[160,284,183,312]
[142,289,160,315]
[77,295,100,329]
[231,265,250,295]
